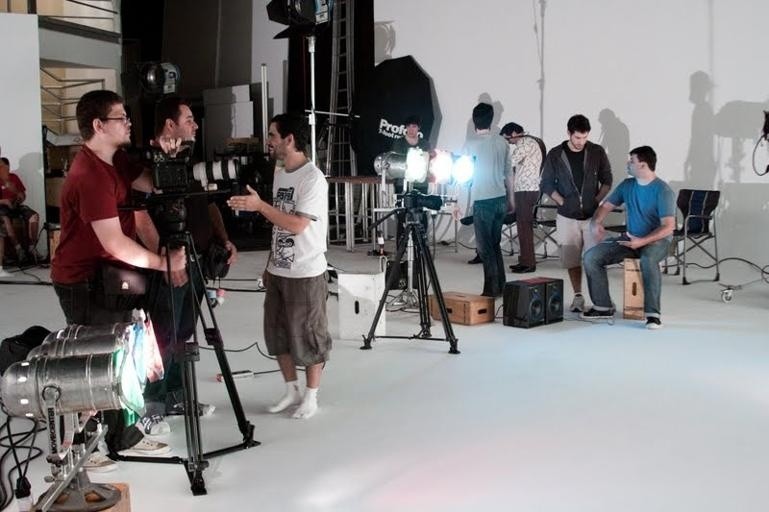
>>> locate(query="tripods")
[359,225,459,355]
[105,233,259,496]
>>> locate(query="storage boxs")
[623,257,645,319]
[46,178,65,207]
[432,290,495,326]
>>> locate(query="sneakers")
[645,319,662,329]
[578,306,615,319]
[17,249,42,260]
[120,436,173,456]
[459,215,473,225]
[141,414,170,434]
[467,256,483,265]
[509,264,536,273]
[165,399,216,418]
[82,451,119,473]
[569,294,586,312]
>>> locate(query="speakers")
[531,276,564,324]
[385,259,408,291]
[503,280,545,328]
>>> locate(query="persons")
[577,146,677,329]
[453,102,515,299]
[392,118,436,263]
[0,157,48,267]
[538,115,615,312]
[50,90,171,474]
[226,113,333,420]
[499,122,544,274]
[129,98,237,435]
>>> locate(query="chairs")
[664,189,720,285]
[0,204,40,270]
[461,179,558,260]
[604,224,627,237]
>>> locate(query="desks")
[325,176,395,250]
[378,204,462,245]
